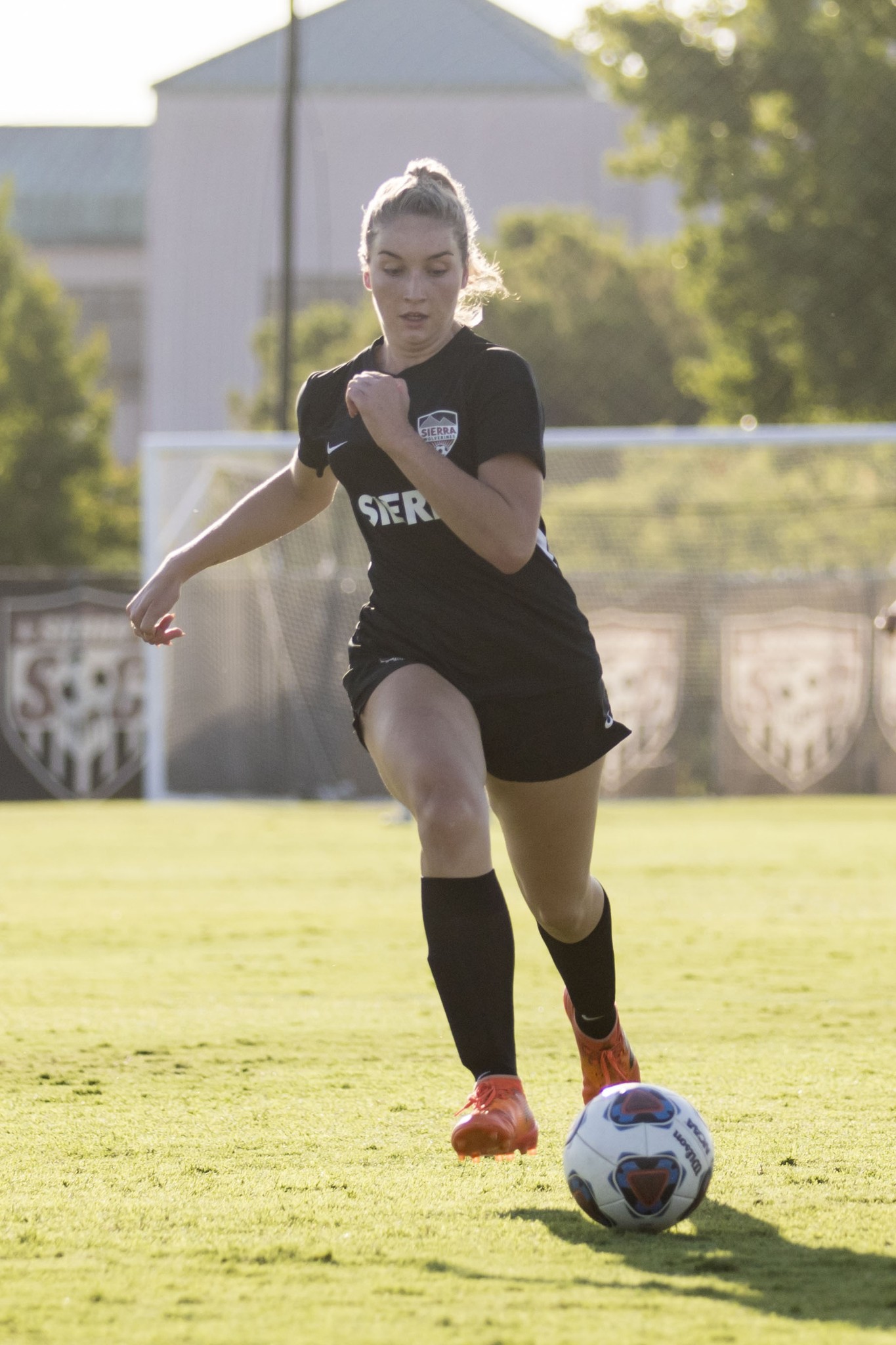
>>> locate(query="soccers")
[562,1082,715,1235]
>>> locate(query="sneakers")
[564,985,643,1107]
[452,1070,538,1159]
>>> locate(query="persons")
[123,159,639,1160]
